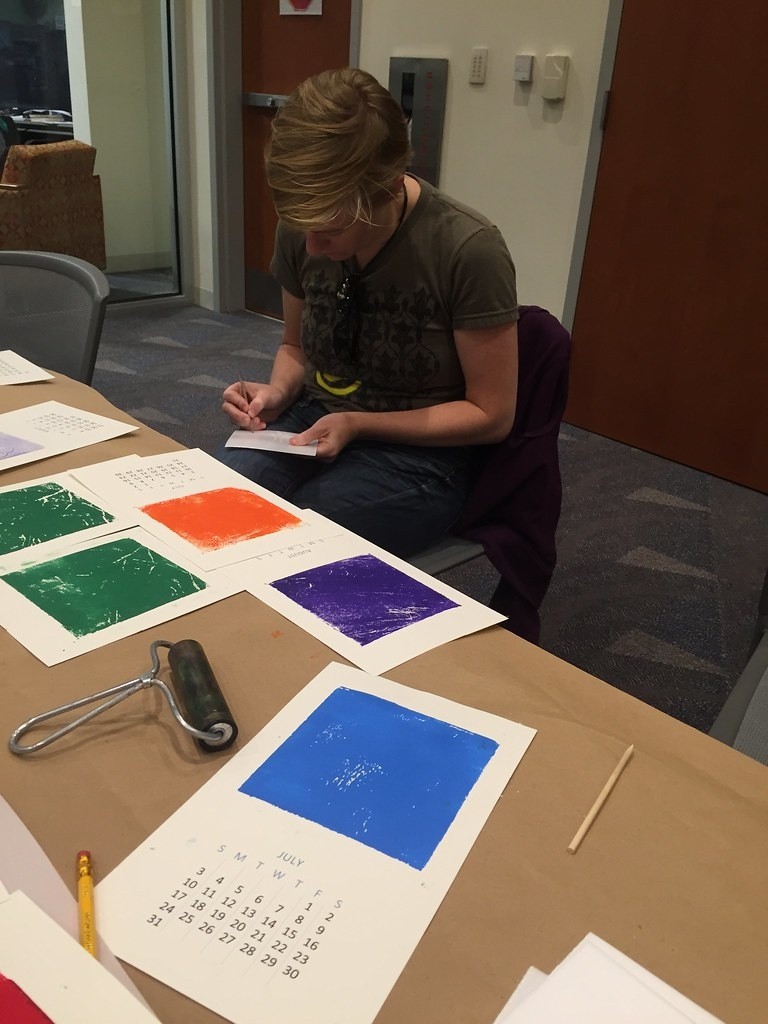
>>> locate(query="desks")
[1,370,767,1023]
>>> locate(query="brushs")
[6,638,237,766]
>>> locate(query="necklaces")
[335,183,409,318]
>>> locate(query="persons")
[207,68,520,555]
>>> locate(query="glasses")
[332,272,364,365]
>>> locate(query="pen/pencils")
[73,849,99,962]
[238,370,254,433]
[567,744,637,857]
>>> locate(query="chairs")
[1,250,109,385]
[0,139,106,269]
[403,304,570,578]
